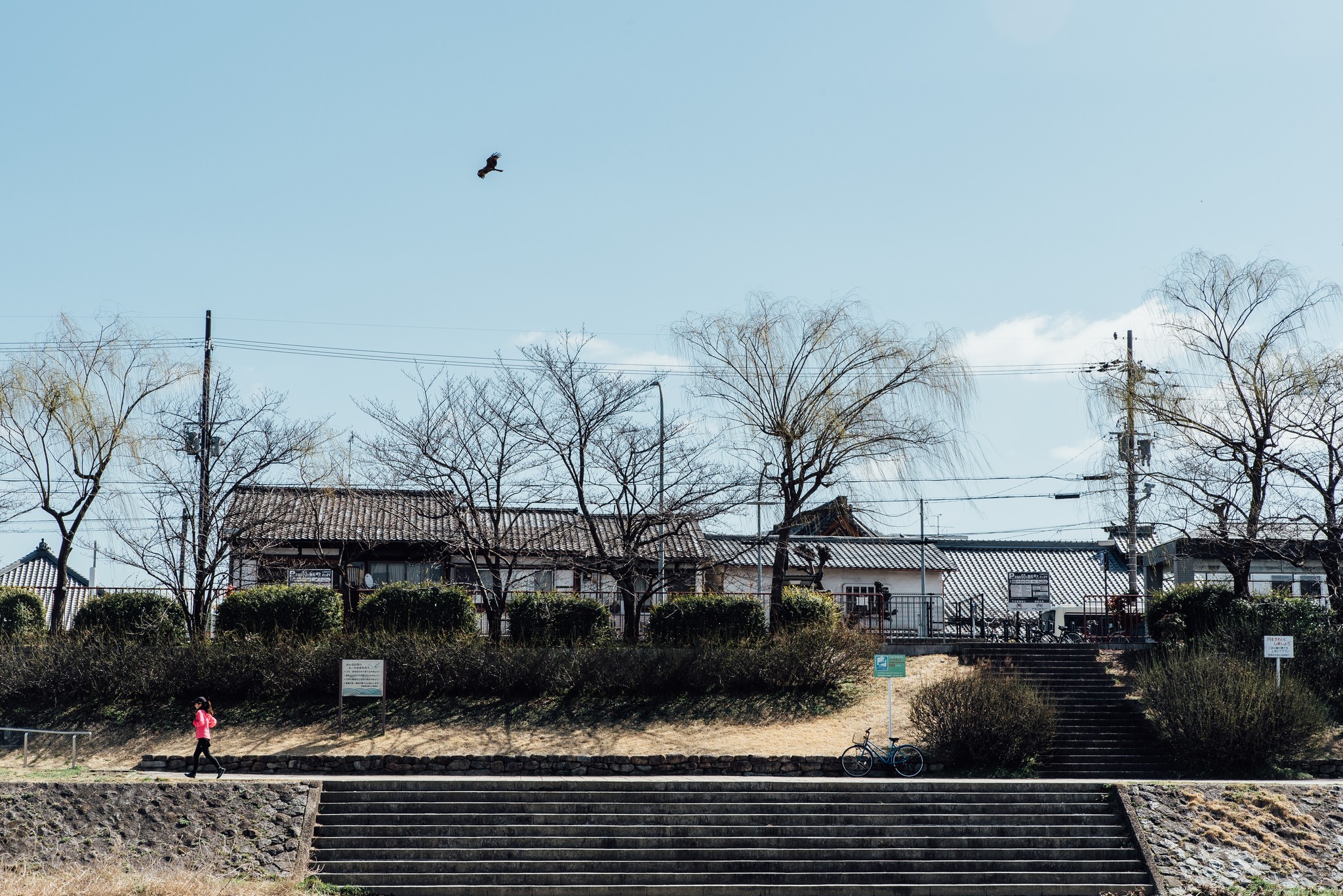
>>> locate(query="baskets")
[852,732,867,746]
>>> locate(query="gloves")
[190,720,194,724]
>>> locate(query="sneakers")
[217,767,226,779]
[184,772,196,778]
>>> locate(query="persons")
[184,697,226,779]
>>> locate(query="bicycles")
[974,614,1130,645]
[841,728,924,778]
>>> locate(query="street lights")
[649,380,665,607]
[757,462,771,605]
[918,499,928,637]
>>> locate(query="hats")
[191,697,207,704]
